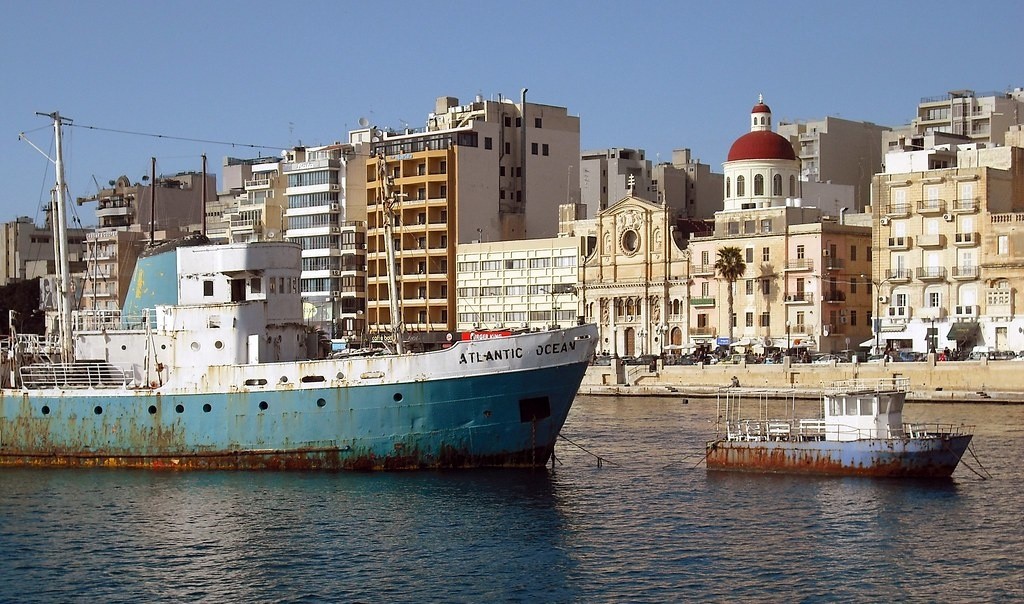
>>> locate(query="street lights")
[784,320,792,356]
[860,273,897,353]
[332,294,341,338]
[655,322,668,359]
[613,326,618,359]
[930,316,936,353]
[307,304,326,327]
[540,287,572,326]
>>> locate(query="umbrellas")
[730,338,757,353]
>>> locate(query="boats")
[0,112,599,469]
[705,377,977,481]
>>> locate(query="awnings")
[946,322,980,341]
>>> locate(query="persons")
[846,351,851,363]
[944,347,950,361]
[771,352,780,364]
[664,351,703,364]
[800,351,812,363]
[752,353,764,361]
[884,348,889,362]
[731,376,740,387]
[722,351,727,358]
[952,348,958,361]
[597,349,611,356]
